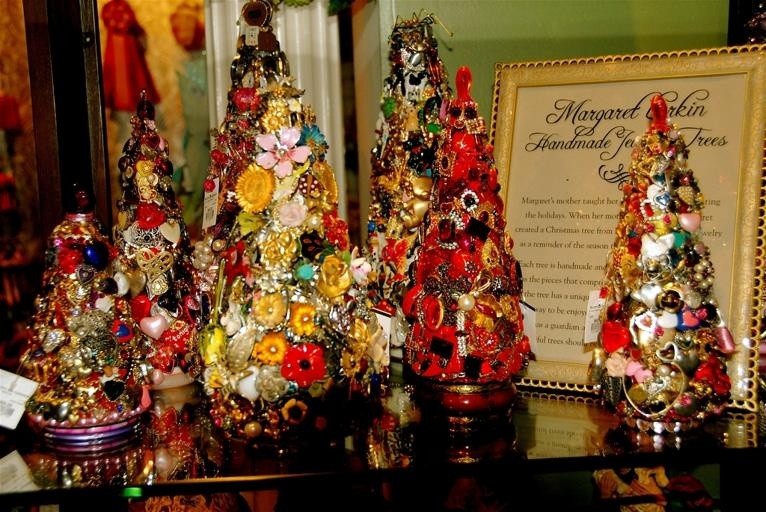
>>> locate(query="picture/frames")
[487,44,766,414]
